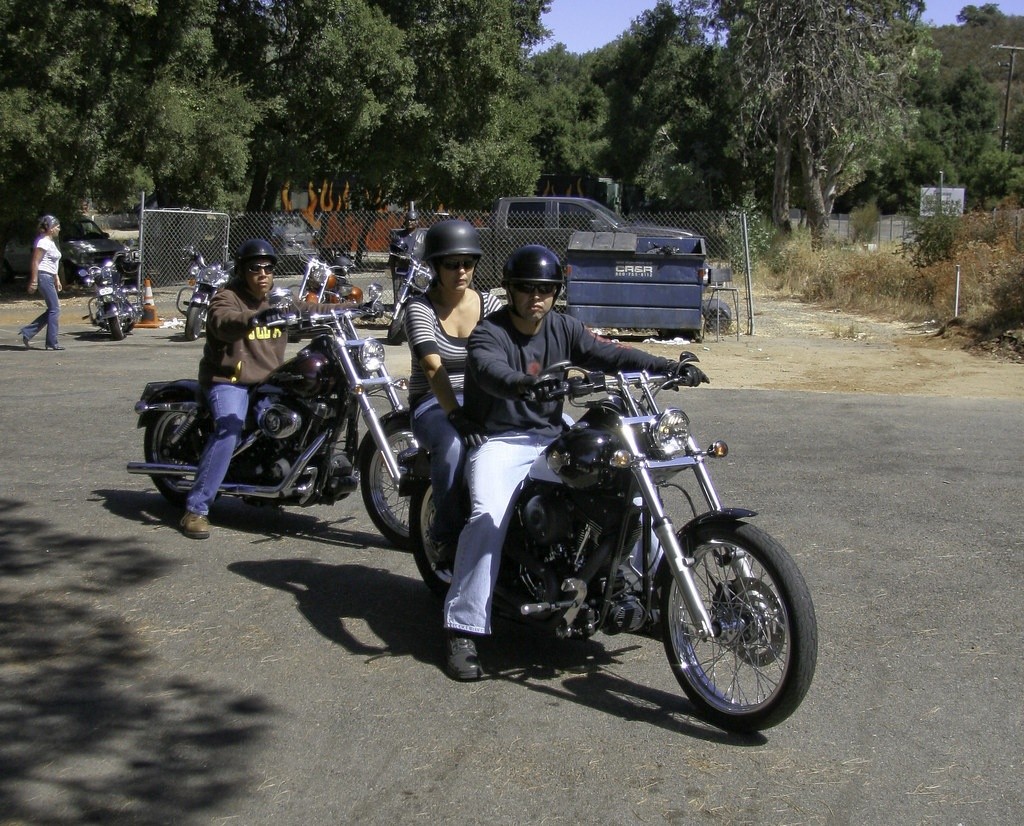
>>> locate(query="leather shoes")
[180,510,209,539]
[445,631,483,679]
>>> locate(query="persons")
[443,245,710,681]
[18,215,66,350]
[389,210,425,309]
[178,239,385,537]
[404,220,502,563]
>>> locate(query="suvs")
[1,219,139,290]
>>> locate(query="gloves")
[519,372,561,402]
[664,359,710,391]
[251,306,286,332]
[360,300,384,322]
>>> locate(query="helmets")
[225,261,236,276]
[236,239,277,271]
[501,244,564,299]
[332,256,350,267]
[121,260,142,273]
[421,220,484,263]
[406,211,420,221]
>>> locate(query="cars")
[215,209,319,274]
[100,208,138,229]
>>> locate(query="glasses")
[433,256,477,272]
[508,281,558,294]
[241,263,276,274]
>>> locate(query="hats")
[40,215,59,232]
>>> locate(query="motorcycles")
[78,252,144,340]
[396,351,819,731]
[282,238,364,305]
[388,246,437,346]
[177,246,237,341]
[127,283,432,552]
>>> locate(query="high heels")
[45,344,66,350]
[18,331,29,348]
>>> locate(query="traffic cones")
[140,280,163,328]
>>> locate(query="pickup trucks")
[388,198,710,311]
